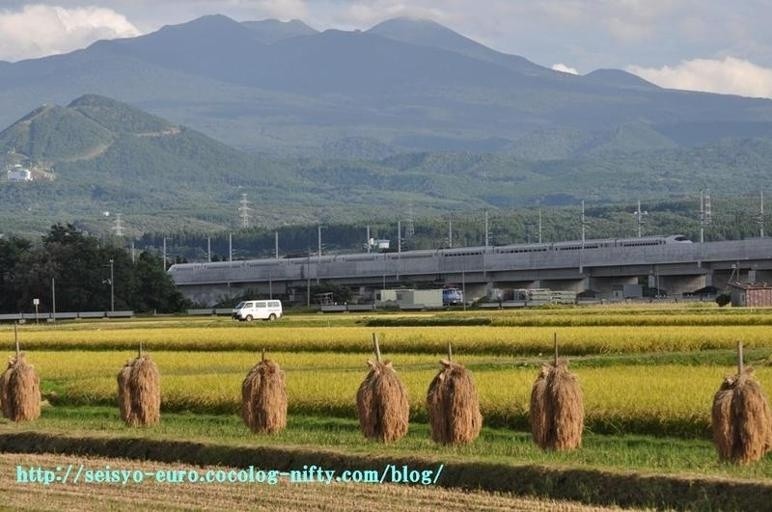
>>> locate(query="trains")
[166,232,696,284]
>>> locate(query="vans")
[231,299,284,323]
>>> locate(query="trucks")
[443,286,464,305]
